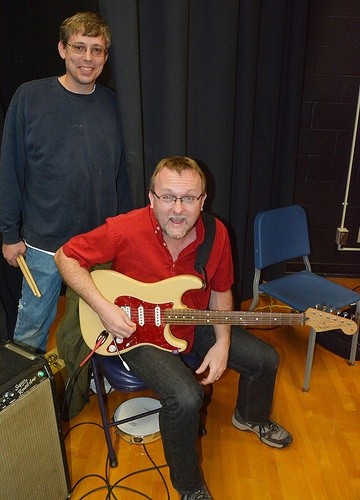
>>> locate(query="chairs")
[55,262,201,468]
[250,205,360,391]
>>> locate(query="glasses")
[152,191,203,205]
[65,42,107,57]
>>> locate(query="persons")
[0,13,133,393]
[54,156,293,500]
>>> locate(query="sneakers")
[232,406,292,449]
[181,482,213,500]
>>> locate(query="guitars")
[78,268,358,357]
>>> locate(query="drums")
[111,396,162,445]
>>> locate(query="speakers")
[0,339,70,500]
